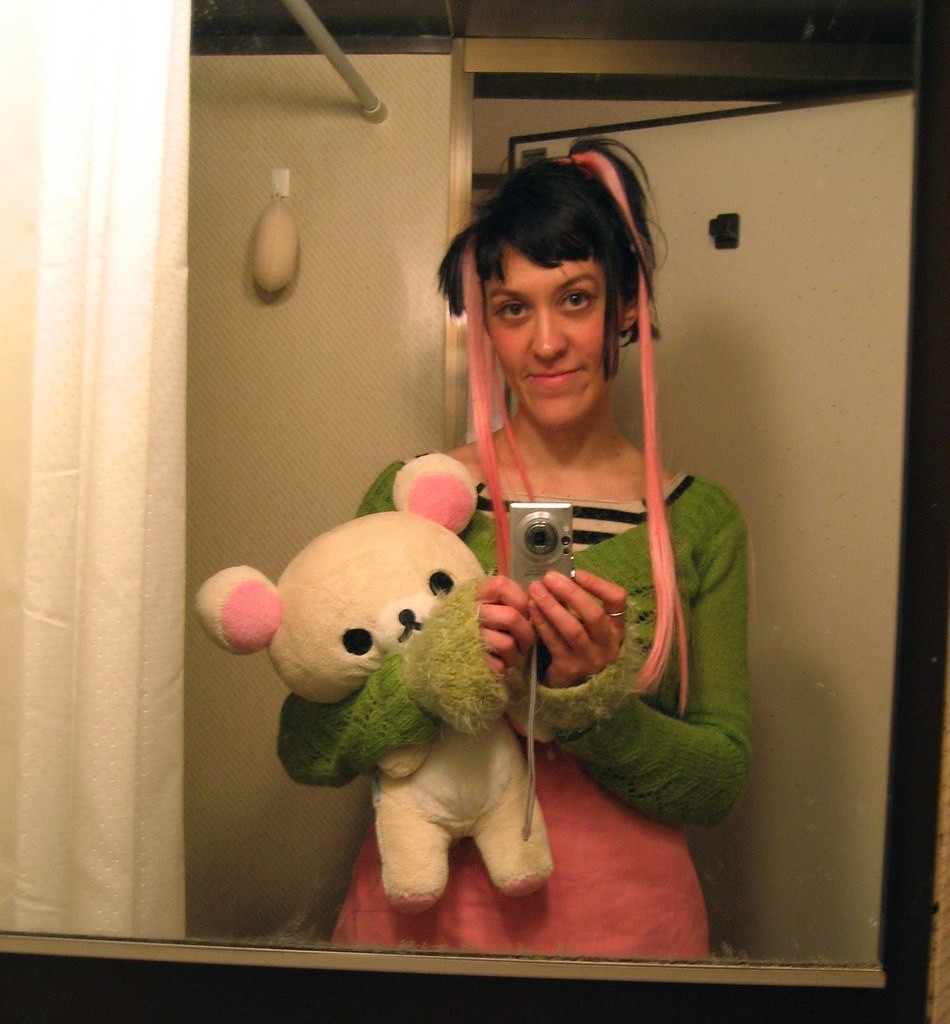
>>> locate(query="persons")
[275,138,753,960]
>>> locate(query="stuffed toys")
[195,454,556,911]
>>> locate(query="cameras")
[509,501,576,599]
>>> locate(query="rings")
[610,610,628,616]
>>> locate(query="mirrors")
[0,0,950,1024]
